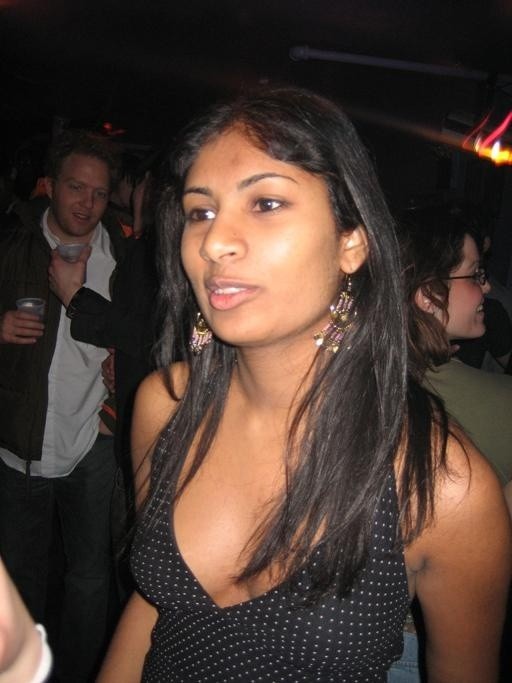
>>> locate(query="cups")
[56,242,87,284]
[16,297,47,324]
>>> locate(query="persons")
[393,211,512,683]
[99,84,512,683]
[1,126,166,683]
